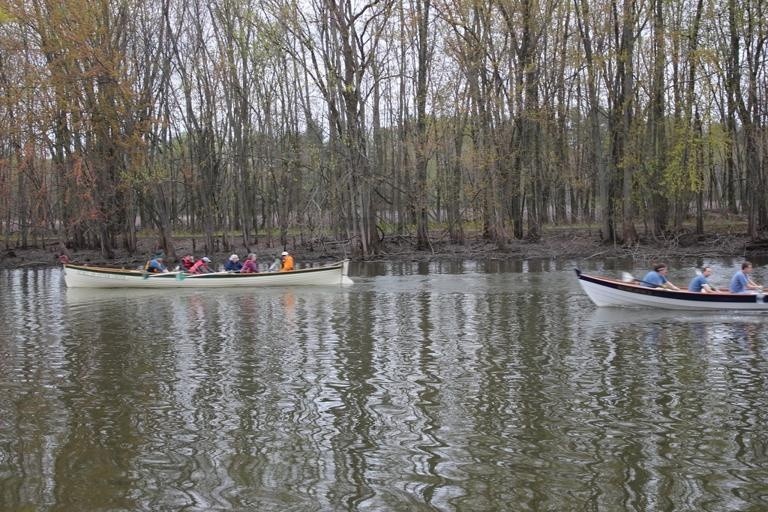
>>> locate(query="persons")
[727,261,764,294]
[639,263,681,291]
[239,253,261,273]
[145,257,168,273]
[188,257,217,274]
[687,266,722,293]
[269,253,282,271]
[278,251,294,272]
[223,254,243,274]
[175,253,195,271]
[56,251,94,267]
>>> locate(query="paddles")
[621,271,663,289]
[177,273,199,280]
[143,271,184,280]
[696,268,718,291]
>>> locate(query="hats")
[281,252,289,256]
[202,257,212,263]
[229,254,240,261]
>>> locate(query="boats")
[56,253,356,289]
[60,284,355,309]
[581,308,768,333]
[571,266,768,313]
